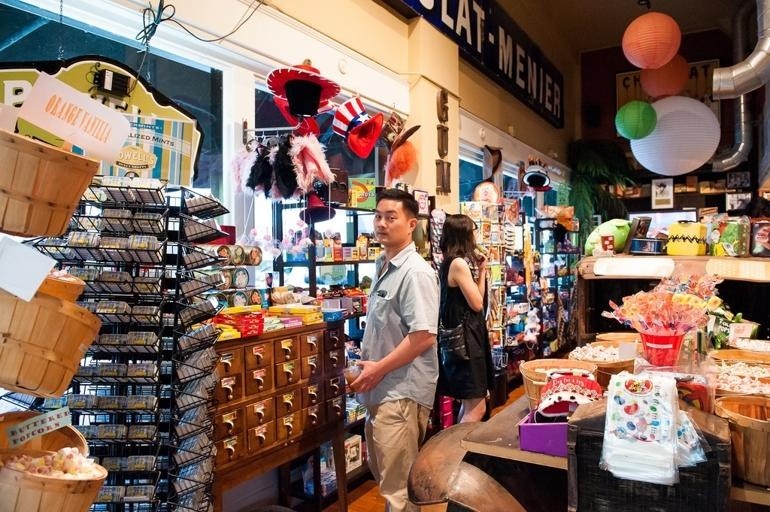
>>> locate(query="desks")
[461,332,768,510]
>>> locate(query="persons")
[250,247,261,265]
[234,246,243,264]
[221,247,227,256]
[435,213,498,426]
[223,271,231,288]
[417,193,426,210]
[347,184,444,512]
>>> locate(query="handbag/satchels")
[438,310,483,367]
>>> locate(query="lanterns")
[628,95,722,178]
[639,53,690,100]
[621,11,683,71]
[614,100,659,142]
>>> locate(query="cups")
[342,366,364,393]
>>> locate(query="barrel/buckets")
[714,394,770,488]
[595,332,641,343]
[519,357,599,412]
[708,347,770,395]
[567,339,644,389]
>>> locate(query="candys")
[601,273,726,336]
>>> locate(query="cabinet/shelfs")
[205,318,345,480]
[193,241,271,296]
[272,198,435,512]
[20,175,230,510]
[570,130,769,337]
[460,202,580,404]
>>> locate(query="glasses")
[472,221,478,231]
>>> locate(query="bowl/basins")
[518,332,642,412]
[704,346,770,489]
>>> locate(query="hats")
[523,164,551,187]
[332,97,384,159]
[266,64,341,102]
[378,112,423,156]
[299,191,336,224]
